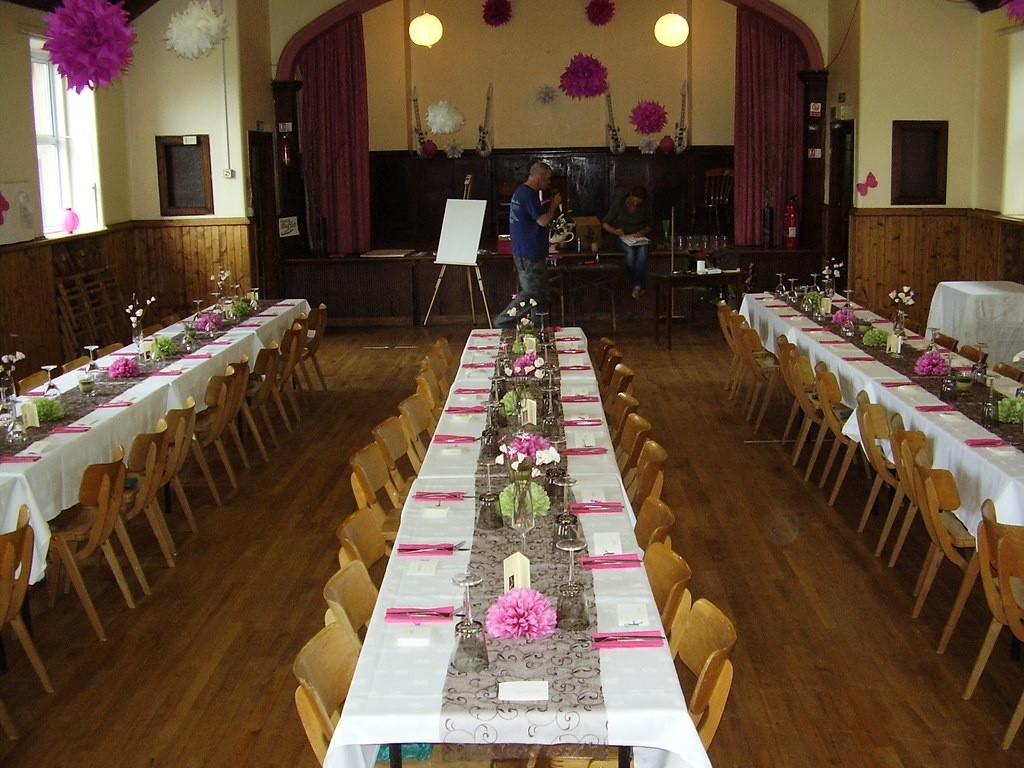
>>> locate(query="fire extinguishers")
[783,194,801,251]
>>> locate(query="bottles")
[576,237,582,254]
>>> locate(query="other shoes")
[632,288,641,298]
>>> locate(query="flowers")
[498,482,552,518]
[494,448,563,478]
[481,592,555,642]
[863,328,891,346]
[123,294,154,330]
[108,357,140,379]
[487,587,558,637]
[197,312,222,331]
[511,334,542,355]
[494,434,557,479]
[500,359,546,387]
[149,336,177,357]
[507,297,541,329]
[916,352,948,376]
[0,351,26,376]
[29,396,64,422]
[833,309,854,325]
[889,288,915,308]
[802,294,824,306]
[998,395,1023,424]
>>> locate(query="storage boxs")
[574,216,602,251]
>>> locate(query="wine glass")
[0,283,260,449]
[773,271,1024,428]
[452,305,590,675]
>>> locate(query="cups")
[677,235,727,251]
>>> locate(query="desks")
[322,329,712,768]
[738,289,1024,594]
[648,269,748,351]
[924,281,1024,368]
[511,260,620,332]
[1,297,311,744]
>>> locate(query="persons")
[602,186,654,301]
[492,162,562,330]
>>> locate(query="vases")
[132,322,144,358]
[0,378,18,409]
[511,379,528,417]
[509,469,536,538]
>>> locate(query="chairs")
[0,300,459,768]
[545,295,1024,768]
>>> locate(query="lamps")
[653,1,688,47]
[409,1,443,47]
